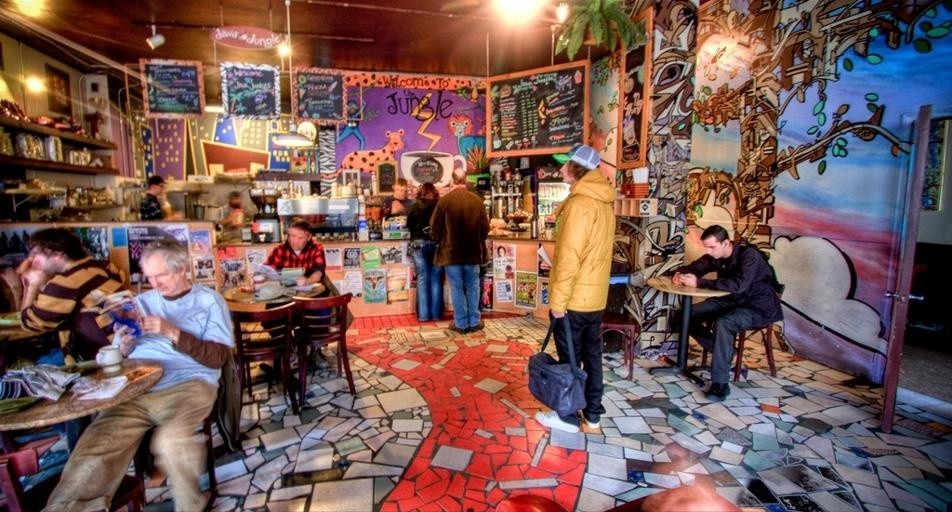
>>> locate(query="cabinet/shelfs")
[0,115,123,223]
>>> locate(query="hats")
[551,142,601,171]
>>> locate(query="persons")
[504,265,514,279]
[380,177,412,222]
[494,245,507,271]
[669,224,780,401]
[428,169,491,333]
[209,191,250,231]
[534,141,619,435]
[19,227,133,367]
[406,182,445,323]
[0,240,55,312]
[41,238,237,512]
[260,220,331,368]
[139,175,184,222]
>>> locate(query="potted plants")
[556,2,647,62]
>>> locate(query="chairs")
[228,301,299,415]
[0,448,143,512]
[133,324,220,512]
[291,292,354,404]
[701,285,786,382]
[600,312,635,380]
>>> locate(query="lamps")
[274,0,313,146]
[146,25,165,50]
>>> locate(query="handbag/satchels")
[527,311,588,418]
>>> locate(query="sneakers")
[447,318,470,334]
[533,409,581,436]
[579,408,601,429]
[467,320,485,332]
[705,381,732,402]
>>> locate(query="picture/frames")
[44,61,73,118]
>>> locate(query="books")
[252,261,305,286]
[91,289,146,345]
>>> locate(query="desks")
[647,276,732,370]
[1,306,48,342]
[223,279,326,393]
[0,360,163,433]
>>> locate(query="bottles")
[503,164,512,181]
[240,272,255,304]
[514,168,521,181]
[531,217,537,239]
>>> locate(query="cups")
[400,150,467,189]
[97,344,123,376]
